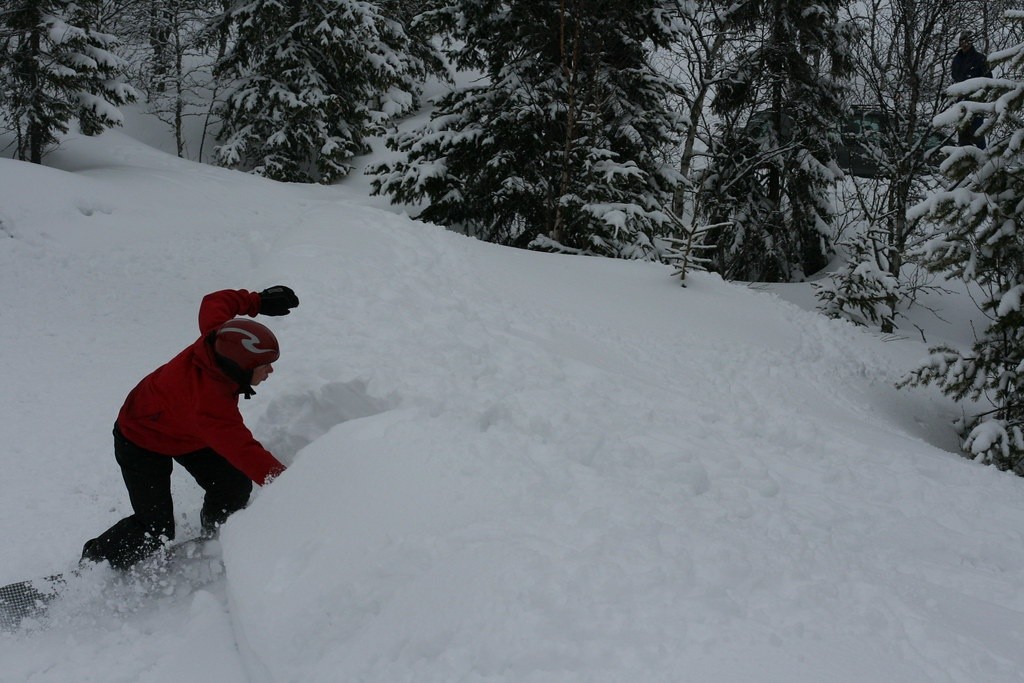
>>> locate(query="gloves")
[259,286,300,316]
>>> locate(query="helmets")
[215,317,281,370]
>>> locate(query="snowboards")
[0,534,225,631]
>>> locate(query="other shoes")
[200,510,217,538]
[78,538,107,571]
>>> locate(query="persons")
[78,285,299,582]
